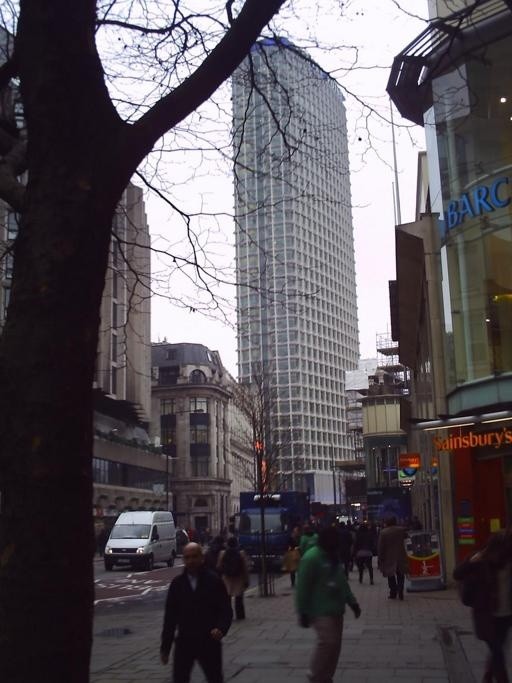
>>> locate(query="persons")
[173,524,254,591]
[96,527,108,557]
[160,541,233,681]
[450,527,511,680]
[282,505,422,600]
[216,535,250,620]
[292,525,361,681]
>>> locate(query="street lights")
[255,436,267,595]
[163,403,208,513]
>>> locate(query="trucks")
[104,508,177,572]
[225,492,322,576]
